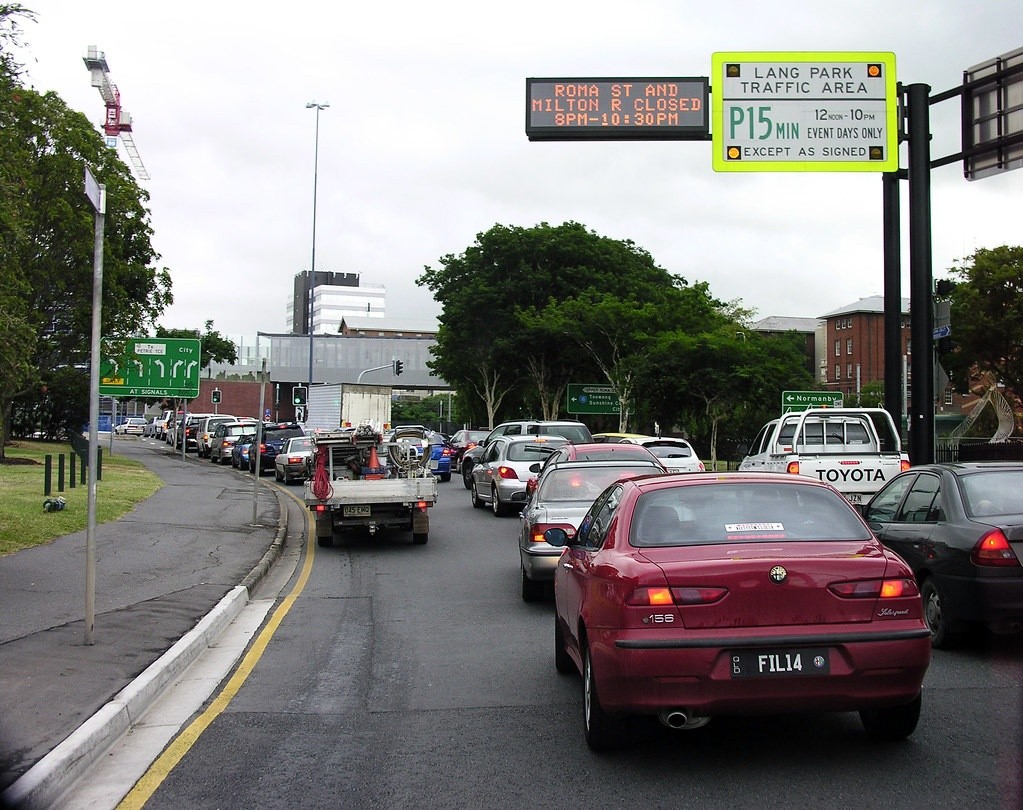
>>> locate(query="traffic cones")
[365,447,384,477]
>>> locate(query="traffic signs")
[782,391,844,415]
[99,336,200,398]
[567,383,635,414]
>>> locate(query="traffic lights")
[293,387,306,405]
[212,390,222,404]
[396,360,404,376]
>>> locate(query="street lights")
[306,100,330,387]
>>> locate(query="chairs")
[641,506,681,538]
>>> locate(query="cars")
[471,434,570,518]
[542,472,933,754]
[115,410,492,486]
[592,433,706,474]
[511,460,698,601]
[851,462,1023,652]
[526,443,669,498]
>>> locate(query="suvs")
[461,418,595,489]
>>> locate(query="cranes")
[82,45,150,181]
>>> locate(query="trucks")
[297,383,393,436]
[737,403,911,505]
[304,431,439,547]
